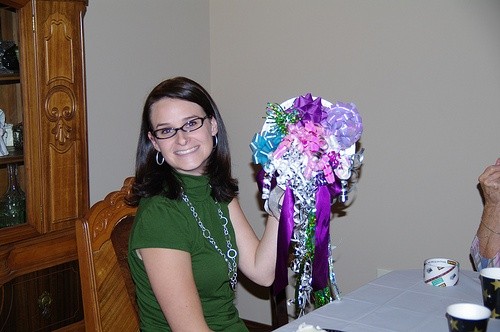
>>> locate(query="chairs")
[75,176,149,332]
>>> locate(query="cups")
[479,268,500,320]
[445,302,493,332]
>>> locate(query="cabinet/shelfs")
[0,0,89,332]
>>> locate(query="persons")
[128,77,285,332]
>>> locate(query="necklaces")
[179,186,237,292]
[470,159,500,273]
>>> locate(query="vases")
[0,164,27,228]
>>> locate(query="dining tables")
[271,269,500,332]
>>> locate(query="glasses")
[151,115,208,139]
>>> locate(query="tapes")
[424,257,460,287]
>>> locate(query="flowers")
[248,92,365,316]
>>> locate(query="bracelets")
[481,220,499,235]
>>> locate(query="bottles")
[0,164,26,227]
